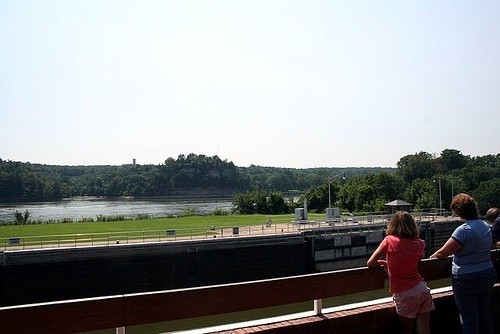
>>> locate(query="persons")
[428,193,496,334]
[367,210,435,334]
[486,207,500,249]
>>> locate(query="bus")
[9,238,19,243]
[166,230,176,235]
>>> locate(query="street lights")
[434,176,441,208]
[329,173,345,208]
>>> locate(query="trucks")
[233,227,239,234]
